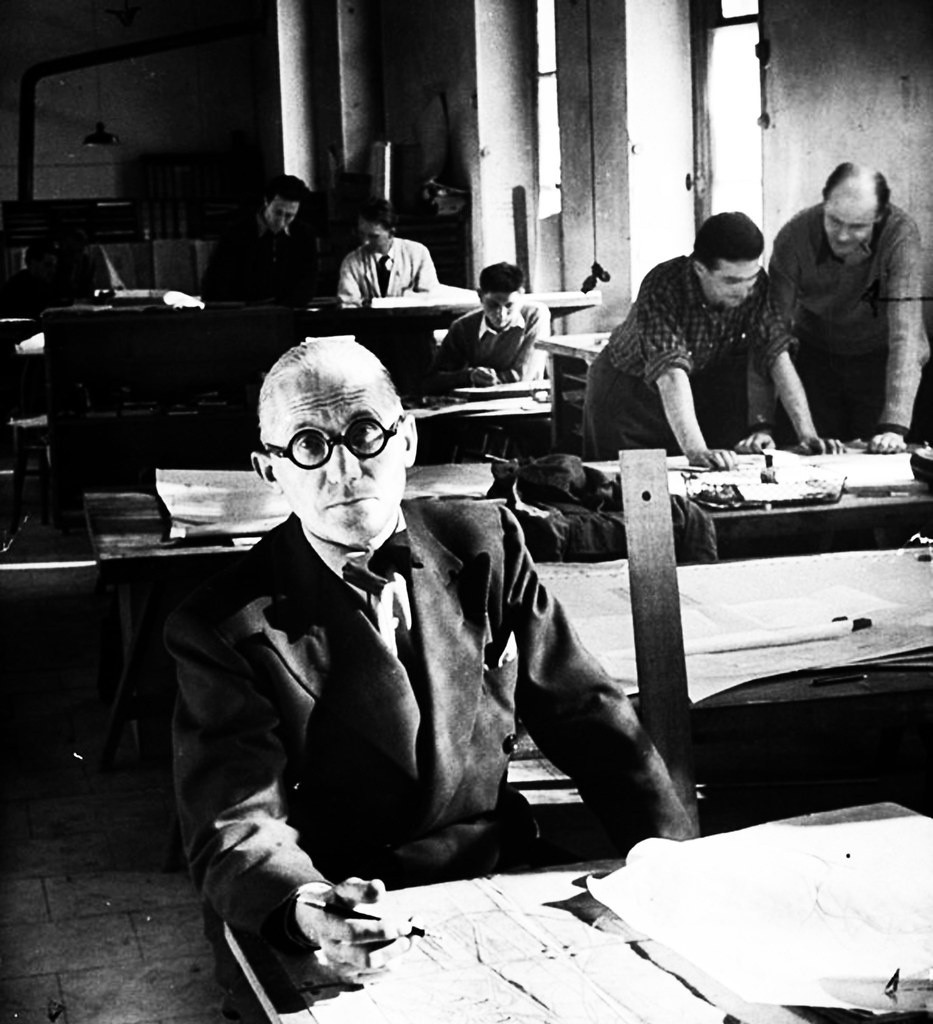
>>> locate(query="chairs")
[9,358,48,536]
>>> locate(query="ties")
[378,255,391,297]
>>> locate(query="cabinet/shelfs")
[545,350,589,453]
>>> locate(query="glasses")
[264,414,407,470]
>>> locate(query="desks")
[414,393,553,464]
[532,544,933,740]
[41,290,600,530]
[83,436,933,761]
[225,798,933,1024]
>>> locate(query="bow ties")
[343,528,423,597]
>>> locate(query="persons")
[0,241,64,318]
[770,161,933,456]
[165,335,697,1024]
[585,211,847,470]
[202,173,313,304]
[427,263,551,385]
[336,194,442,309]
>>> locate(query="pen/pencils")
[468,367,503,385]
[303,898,426,939]
